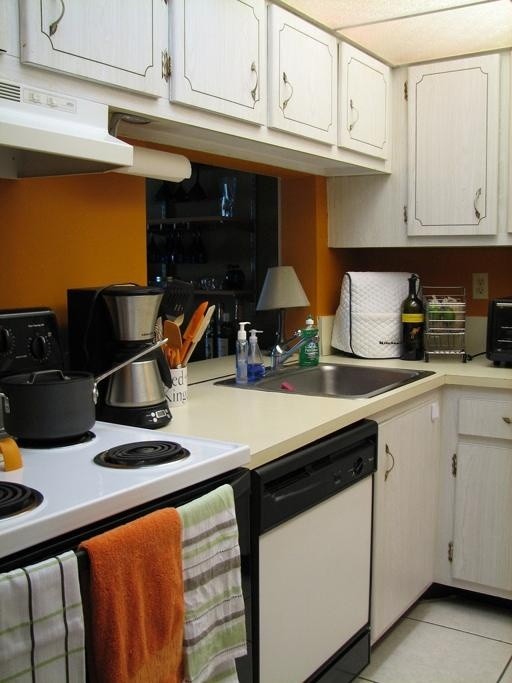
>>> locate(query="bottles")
[401,274,427,360]
[202,302,244,359]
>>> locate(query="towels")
[1,549,87,682]
[78,507,186,682]
[174,482,248,682]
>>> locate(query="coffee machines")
[63,284,175,429]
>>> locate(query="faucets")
[272,333,319,376]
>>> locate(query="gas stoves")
[0,417,250,534]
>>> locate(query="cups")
[164,366,189,407]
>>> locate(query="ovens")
[0,471,256,682]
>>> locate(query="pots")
[1,335,169,438]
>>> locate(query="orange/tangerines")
[429,307,440,322]
[440,307,455,323]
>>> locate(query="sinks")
[214,362,436,398]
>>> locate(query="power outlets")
[471,272,490,302]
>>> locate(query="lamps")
[255,265,311,358]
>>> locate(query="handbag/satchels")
[329,269,423,360]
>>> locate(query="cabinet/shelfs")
[167,0,337,179]
[1,0,166,121]
[337,41,392,174]
[443,382,512,603]
[355,385,443,648]
[402,49,512,247]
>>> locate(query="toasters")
[485,297,512,366]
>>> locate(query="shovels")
[162,319,185,368]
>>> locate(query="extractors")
[0,79,136,179]
